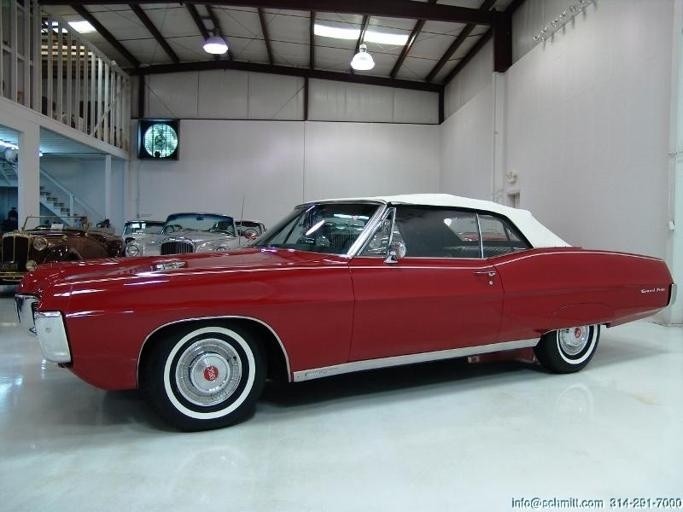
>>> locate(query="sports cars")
[0,215,122,287]
[13,192,677,432]
[126,211,249,258]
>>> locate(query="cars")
[215,219,265,241]
[121,220,164,243]
[455,231,514,241]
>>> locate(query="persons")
[8,207,18,222]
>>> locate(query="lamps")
[350,40,375,71]
[202,25,227,55]
